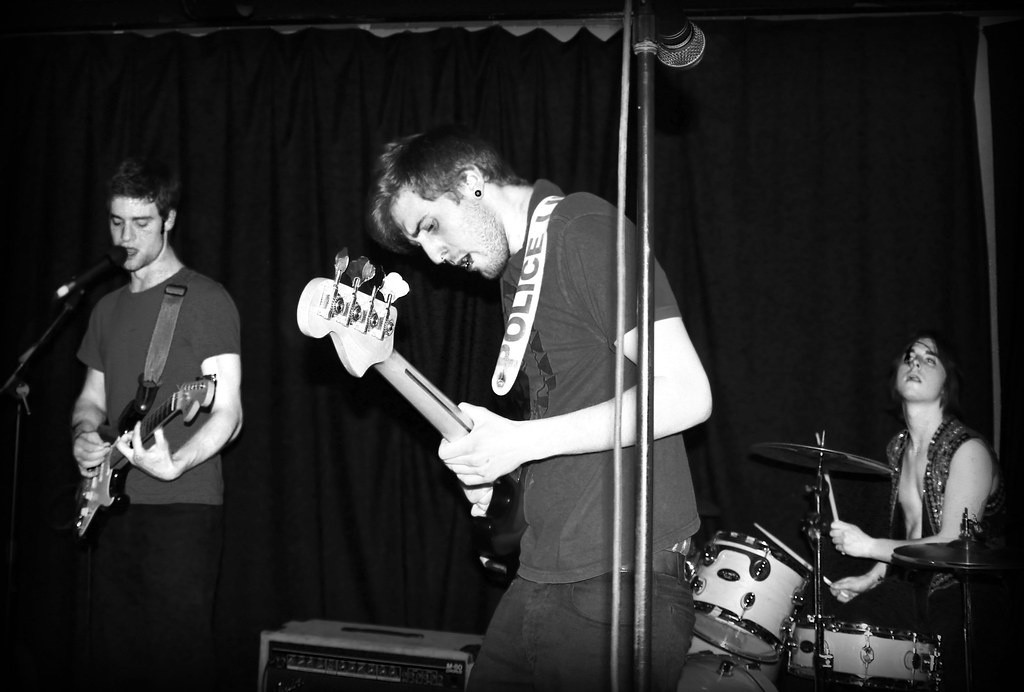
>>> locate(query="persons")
[366,129,712,692]
[70,157,243,691]
[829,329,1006,634]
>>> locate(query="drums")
[785,614,944,692]
[687,528,813,684]
[676,649,780,692]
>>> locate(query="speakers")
[258,618,485,692]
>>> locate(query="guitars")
[73,374,219,550]
[295,246,525,586]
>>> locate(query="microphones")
[651,0,706,69]
[57,245,128,298]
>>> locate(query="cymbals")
[892,537,1024,570]
[747,441,895,476]
